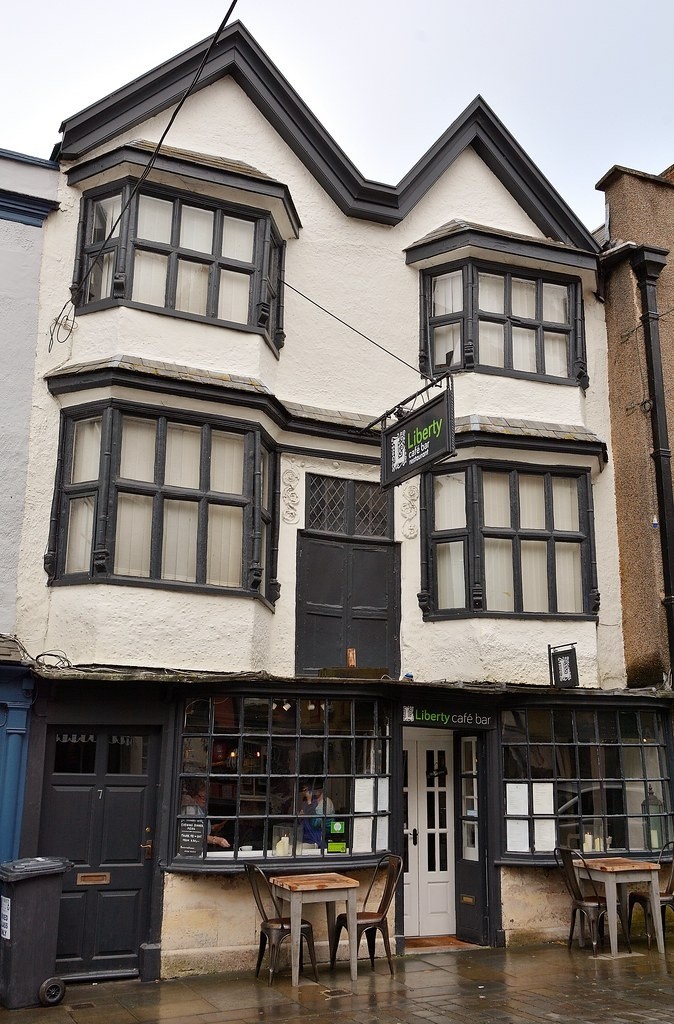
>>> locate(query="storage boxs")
[209,783,223,798]
[222,784,236,799]
[234,784,252,799]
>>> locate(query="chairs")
[629,841,674,950]
[243,860,320,986]
[328,854,404,976]
[554,847,632,958]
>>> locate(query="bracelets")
[212,836,217,844]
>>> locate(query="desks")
[269,872,361,986]
[572,857,666,958]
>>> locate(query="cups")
[239,846,252,851]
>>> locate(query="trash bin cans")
[0,855,75,1009]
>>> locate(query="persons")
[179,777,231,852]
[297,778,335,850]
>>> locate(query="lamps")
[307,700,315,711]
[320,704,324,710]
[283,699,291,711]
[273,703,277,710]
